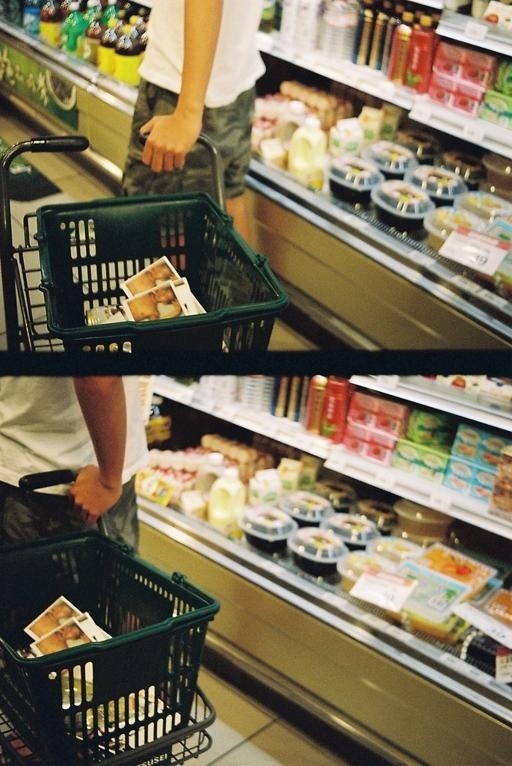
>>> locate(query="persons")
[2,374,148,563]
[35,622,84,656]
[25,600,75,640]
[126,261,174,298]
[127,286,176,322]
[117,0,267,250]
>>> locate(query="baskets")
[33,192,289,354]
[0,529,221,766]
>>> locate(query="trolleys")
[0,470,220,766]
[2,132,290,353]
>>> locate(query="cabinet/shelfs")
[0,0,511,352]
[134,368,511,765]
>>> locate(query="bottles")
[355,0,440,96]
[0,0,150,87]
[272,374,350,445]
